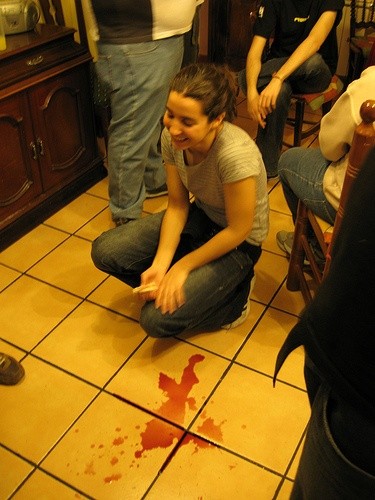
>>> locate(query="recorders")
[0,0,42,36]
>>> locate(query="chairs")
[285,100,375,302]
[256,4,343,148]
[345,0,375,88]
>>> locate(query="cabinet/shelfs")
[0,21,106,253]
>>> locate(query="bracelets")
[273,72,283,83]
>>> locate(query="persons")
[0,349,25,386]
[239,0,346,175]
[91,61,270,339]
[272,140,375,500]
[276,65,375,266]
[88,0,206,227]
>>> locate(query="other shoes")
[116,217,136,227]
[220,273,256,329]
[0,352,25,385]
[146,183,168,198]
[276,228,295,253]
[284,237,311,265]
[267,172,278,178]
